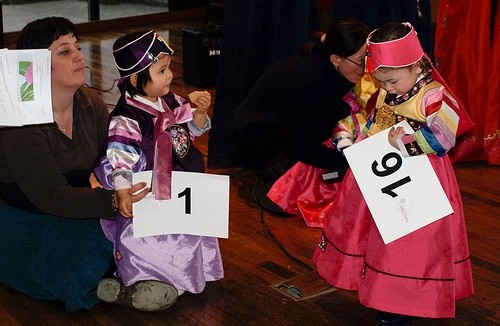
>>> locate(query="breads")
[188,90,208,103]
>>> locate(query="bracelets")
[111,194,116,211]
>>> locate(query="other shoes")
[97,278,178,310]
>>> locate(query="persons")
[0,15,180,314]
[89,29,225,297]
[225,17,474,326]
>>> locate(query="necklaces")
[56,119,66,134]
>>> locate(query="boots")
[249,147,292,213]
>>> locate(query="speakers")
[183,27,219,88]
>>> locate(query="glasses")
[342,56,365,70]
